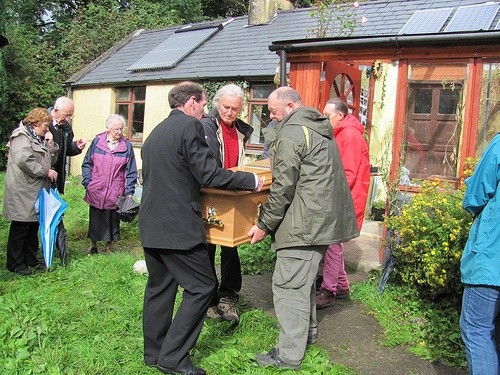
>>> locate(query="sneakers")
[206,306,225,322]
[217,297,240,324]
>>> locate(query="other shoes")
[157,359,206,375]
[275,324,316,343]
[335,288,349,299]
[29,261,47,270]
[102,248,112,255]
[255,348,300,369]
[316,289,335,309]
[8,267,32,275]
[85,247,99,255]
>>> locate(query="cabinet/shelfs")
[198,158,277,248]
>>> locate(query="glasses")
[59,110,75,120]
[113,128,124,134]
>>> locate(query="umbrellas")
[115,194,141,222]
[34,186,67,271]
[49,178,68,268]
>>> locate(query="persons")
[4,107,59,276]
[247,87,360,369]
[199,85,253,322]
[82,114,138,256]
[313,98,369,309]
[140,83,264,375]
[457,103,500,375]
[36,96,86,251]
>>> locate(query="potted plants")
[370,200,385,221]
